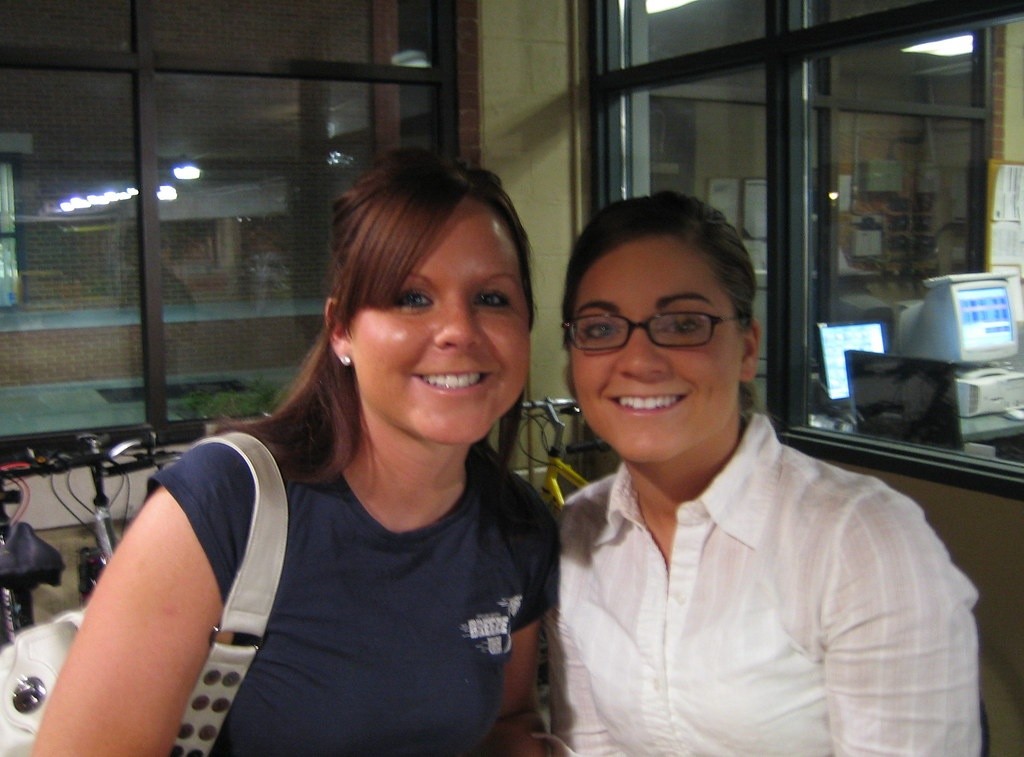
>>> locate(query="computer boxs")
[955,371,1024,419]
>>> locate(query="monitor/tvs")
[813,319,890,405]
[844,350,964,451]
[900,279,1018,378]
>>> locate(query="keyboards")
[923,269,1019,287]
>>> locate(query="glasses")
[560,310,751,351]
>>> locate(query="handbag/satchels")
[0,431,288,757]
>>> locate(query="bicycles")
[519,396,599,512]
[0,423,163,644]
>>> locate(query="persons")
[28,146,560,757]
[536,188,984,757]
